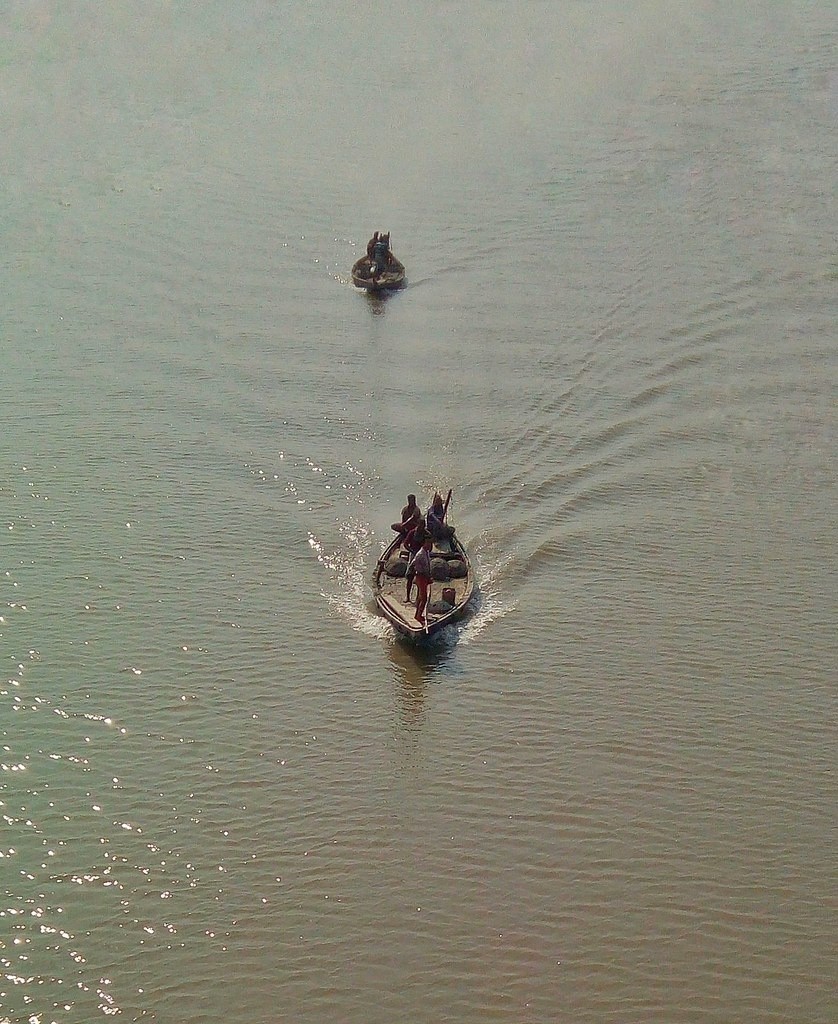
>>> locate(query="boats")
[372,505,475,640]
[351,240,405,296]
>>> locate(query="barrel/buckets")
[442,587,457,605]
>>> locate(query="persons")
[391,494,426,539]
[367,231,390,283]
[427,495,455,540]
[404,517,433,622]
